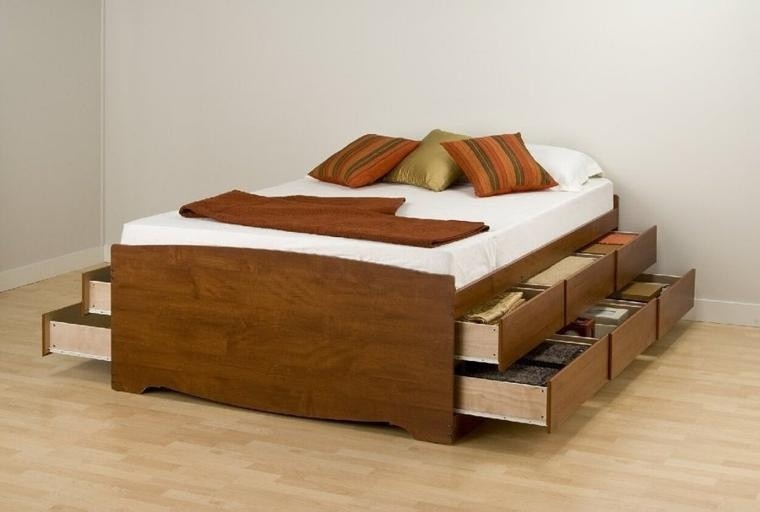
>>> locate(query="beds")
[38,127,697,447]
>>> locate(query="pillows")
[306,130,561,198]
